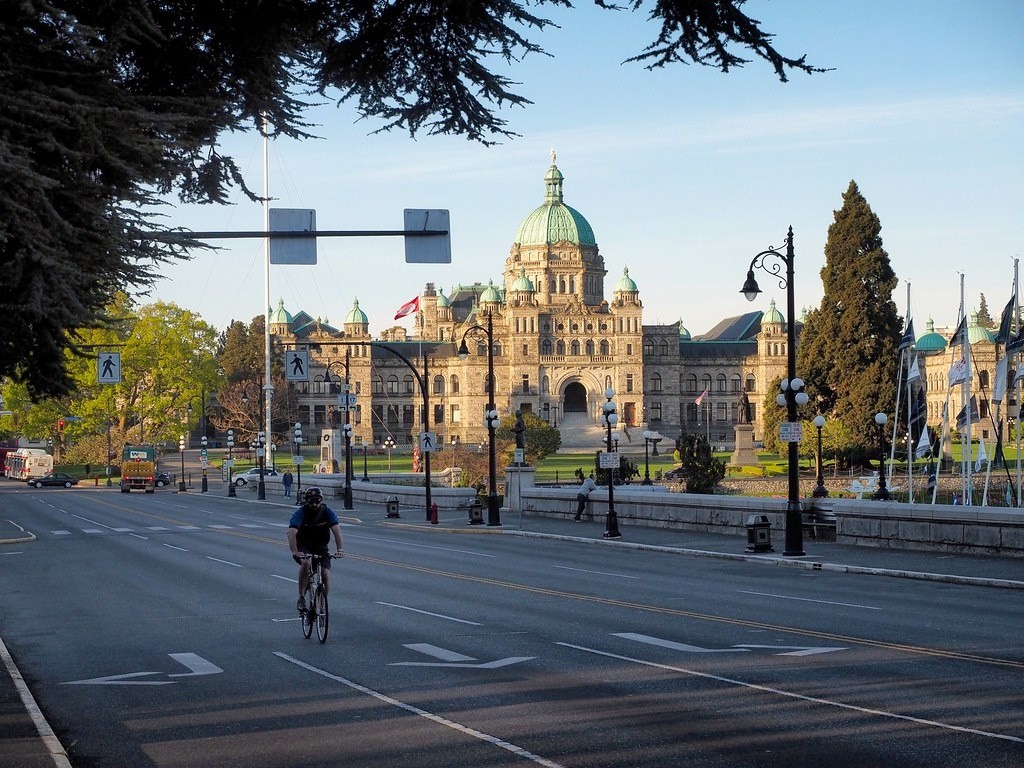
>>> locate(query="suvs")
[231,468,278,487]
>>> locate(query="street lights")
[362,441,370,482]
[272,444,278,476]
[292,423,305,505]
[187,395,208,493]
[180,435,187,491]
[599,389,623,539]
[241,382,266,499]
[740,251,806,555]
[813,417,828,496]
[457,327,503,526]
[641,431,653,485]
[226,430,237,497]
[382,437,396,473]
[322,361,353,510]
[875,413,892,500]
[83,392,112,487]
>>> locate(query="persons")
[287,487,343,619]
[738,392,751,423]
[316,316,321,329]
[282,469,293,499]
[633,462,640,477]
[85,462,92,479]
[572,474,597,522]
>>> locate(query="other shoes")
[319,610,327,621]
[285,497,290,500]
[297,596,308,611]
[576,520,582,522]
[573,518,576,521]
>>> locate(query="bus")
[0,448,54,482]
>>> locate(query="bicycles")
[298,553,342,644]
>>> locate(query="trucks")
[120,444,156,493]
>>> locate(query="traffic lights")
[58,420,65,432]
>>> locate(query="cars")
[664,466,708,481]
[27,472,79,489]
[156,472,170,487]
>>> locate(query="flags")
[394,296,419,320]
[695,387,708,406]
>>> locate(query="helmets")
[303,487,323,500]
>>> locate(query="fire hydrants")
[95,475,99,485]
[431,503,440,524]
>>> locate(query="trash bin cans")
[384,495,401,518]
[466,498,485,525]
[746,515,775,552]
[313,459,340,474]
[296,489,306,506]
[655,471,661,480]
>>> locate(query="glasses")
[308,500,322,504]
